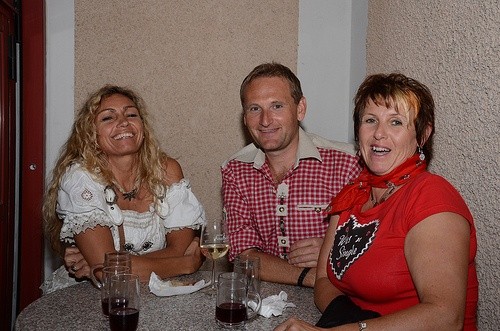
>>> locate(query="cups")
[100,266,131,316]
[232,255,260,307]
[90,251,132,293]
[215,272,262,327]
[108,274,140,331]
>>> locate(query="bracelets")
[358,320,367,331]
[297,267,312,287]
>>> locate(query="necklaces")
[369,184,395,208]
[113,174,142,201]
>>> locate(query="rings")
[71,265,80,272]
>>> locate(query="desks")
[15,269,323,331]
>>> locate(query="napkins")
[243,290,296,319]
[149,271,211,297]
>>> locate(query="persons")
[40,84,207,296]
[273,70,481,331]
[221,62,364,287]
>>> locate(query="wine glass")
[200,219,231,296]
